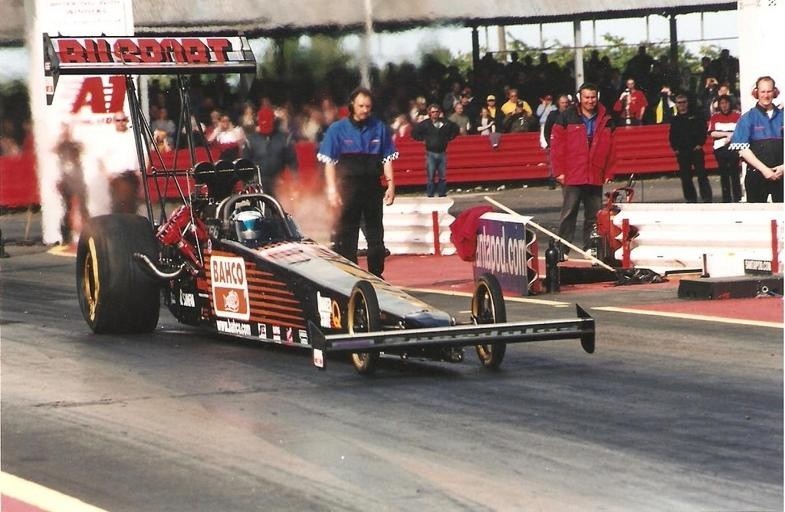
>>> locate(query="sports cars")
[36,29,606,375]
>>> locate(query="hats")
[259,109,274,133]
[486,94,496,101]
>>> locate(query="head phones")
[575,82,601,103]
[752,76,780,99]
[349,87,377,113]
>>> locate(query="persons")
[148,58,362,198]
[586,47,741,126]
[98,111,150,177]
[229,209,271,243]
[376,47,576,197]
[667,75,784,201]
[49,121,91,247]
[315,86,400,282]
[549,83,617,255]
